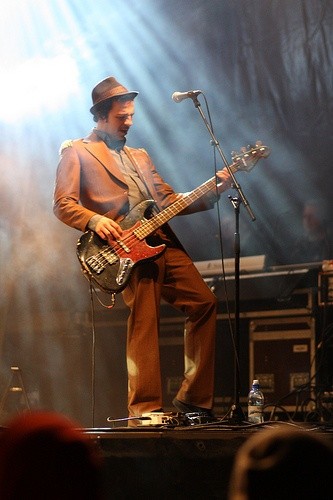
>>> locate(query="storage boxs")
[249,317,325,412]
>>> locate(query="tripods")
[189,97,265,431]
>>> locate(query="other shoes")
[171,398,218,423]
[127,414,141,428]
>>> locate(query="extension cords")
[141,413,175,425]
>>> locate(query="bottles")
[248,380,264,424]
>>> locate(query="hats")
[89,76,139,114]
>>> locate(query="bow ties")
[104,134,126,153]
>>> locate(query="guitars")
[77,141,272,294]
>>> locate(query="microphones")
[172,90,202,103]
